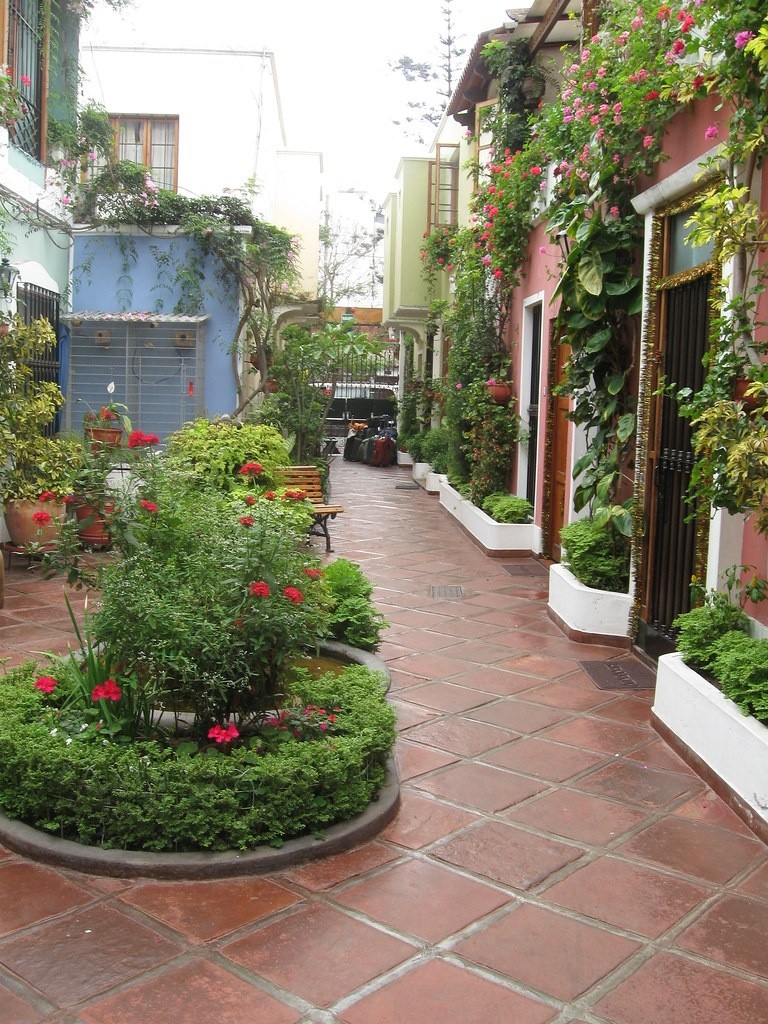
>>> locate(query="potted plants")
[480,35,560,108]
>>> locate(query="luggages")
[343,415,397,466]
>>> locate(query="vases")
[85,426,126,451]
[72,638,389,733]
[70,497,125,544]
[0,431,85,548]
[487,384,512,405]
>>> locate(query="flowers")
[74,381,132,437]
[91,437,338,707]
[487,376,512,385]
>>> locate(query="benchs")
[272,464,342,551]
